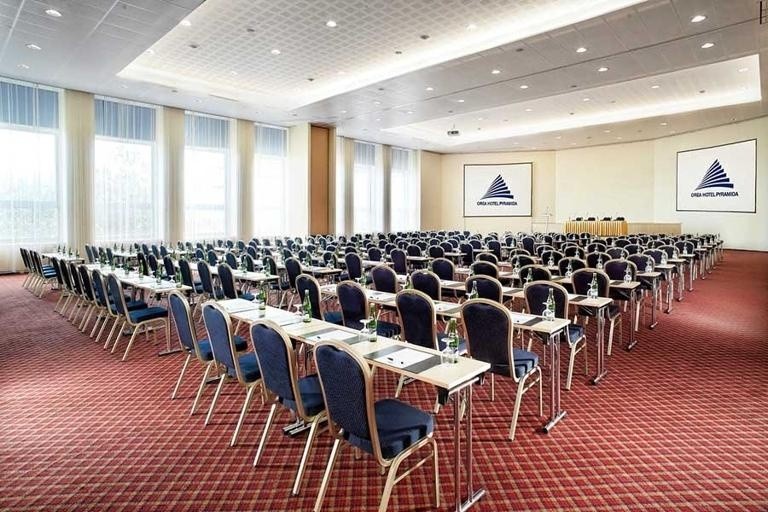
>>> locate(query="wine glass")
[292,302,303,324]
[439,337,456,368]
[250,293,260,309]
[358,319,373,343]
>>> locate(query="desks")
[205,295,497,509]
[315,282,572,434]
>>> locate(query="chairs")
[15,215,725,390]
[201,302,265,444]
[306,345,458,508]
[454,294,546,439]
[245,322,331,499]
[165,290,249,414]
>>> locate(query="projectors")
[447,129,460,136]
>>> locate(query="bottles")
[368,302,378,342]
[250,230,510,300]
[258,280,266,312]
[449,319,459,363]
[510,233,683,298]
[545,286,556,319]
[303,289,313,323]
[55,228,249,288]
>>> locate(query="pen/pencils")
[317,336,322,339]
[388,357,403,364]
